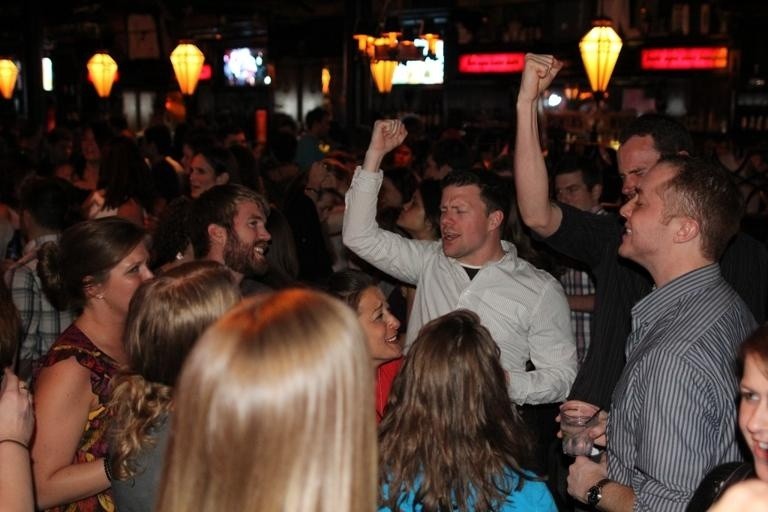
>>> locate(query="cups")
[559,400,600,457]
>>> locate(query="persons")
[0,52,768,511]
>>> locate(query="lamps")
[0,0,18,99]
[369,0,398,96]
[352,0,439,66]
[84,0,119,98]
[170,1,206,96]
[577,1,624,103]
